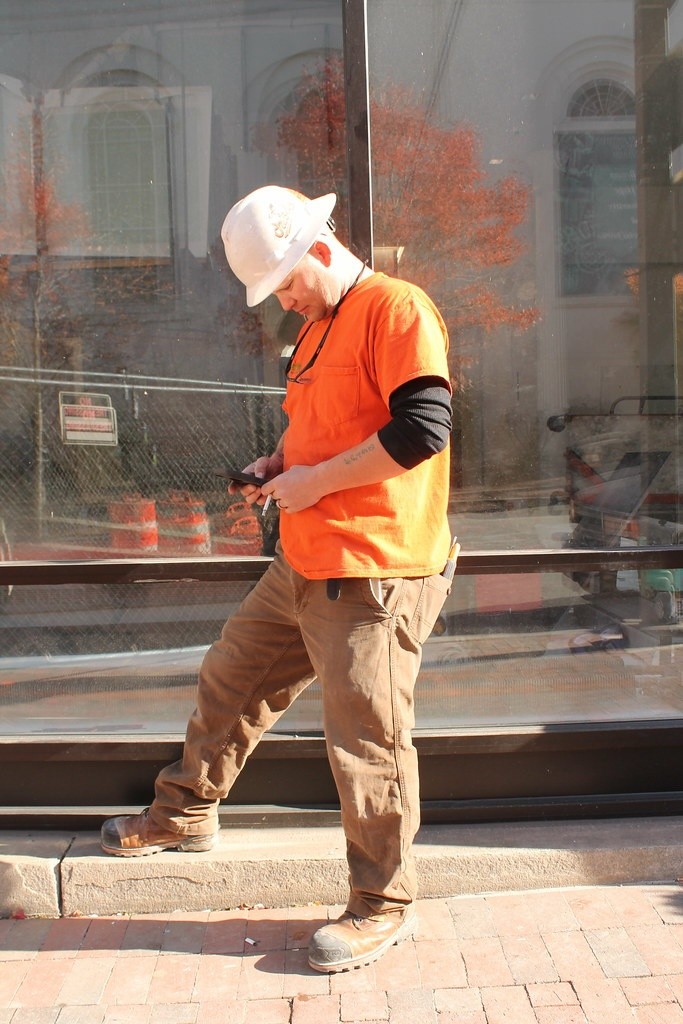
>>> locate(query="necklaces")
[284,257,370,384]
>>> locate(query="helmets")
[220,185,338,308]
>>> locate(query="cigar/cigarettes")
[262,493,272,517]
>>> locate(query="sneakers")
[308,894,417,974]
[100,807,219,858]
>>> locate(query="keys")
[327,579,341,600]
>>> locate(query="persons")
[100,185,459,974]
